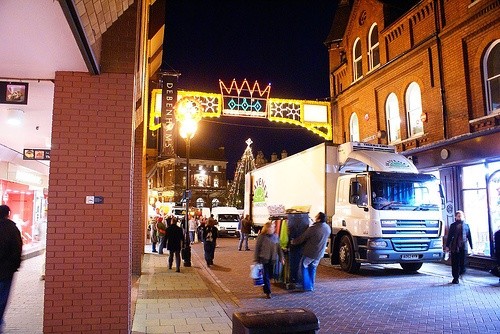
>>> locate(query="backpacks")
[205,226,213,242]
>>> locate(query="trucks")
[244,141,445,275]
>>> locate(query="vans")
[169,208,211,219]
[212,206,241,238]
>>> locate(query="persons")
[201,217,219,266]
[289,211,330,292]
[238,213,254,250]
[0,204,23,329]
[149,207,219,254]
[163,218,186,272]
[446,210,473,284]
[253,221,285,300]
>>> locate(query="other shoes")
[452,277,459,284]
[267,293,272,299]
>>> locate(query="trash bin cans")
[232,307,320,334]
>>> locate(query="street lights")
[178,119,197,267]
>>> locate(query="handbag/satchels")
[449,237,460,253]
[181,248,186,259]
[249,263,265,287]
